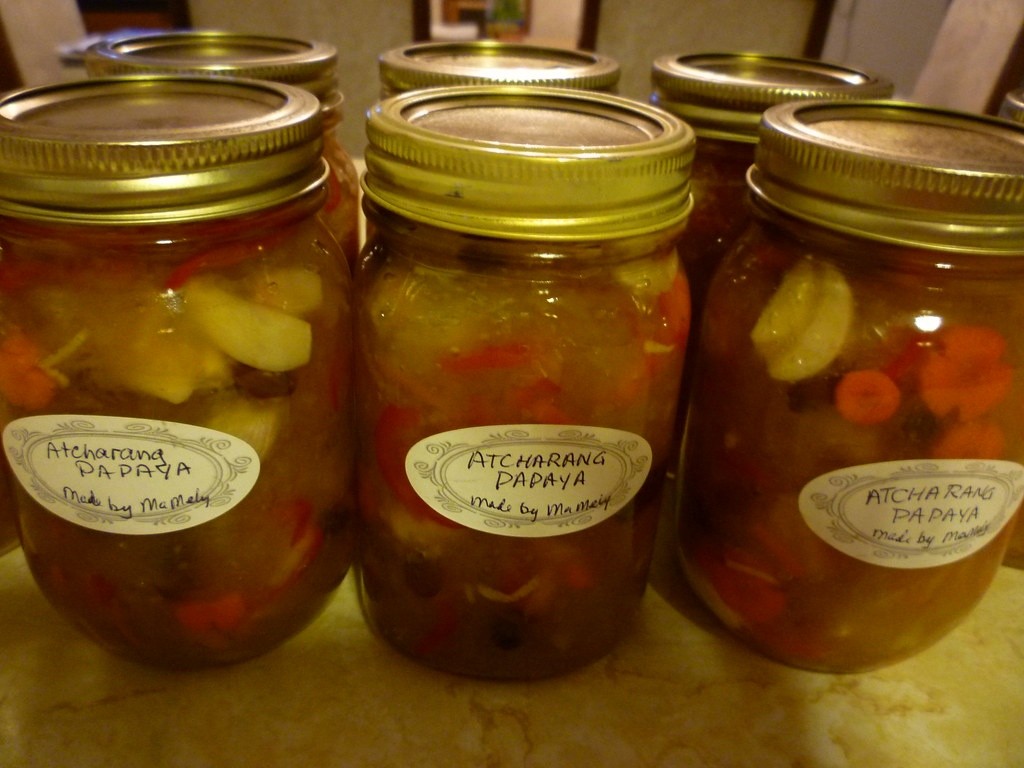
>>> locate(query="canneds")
[0,25,1017,680]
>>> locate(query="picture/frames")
[408,2,600,61]
